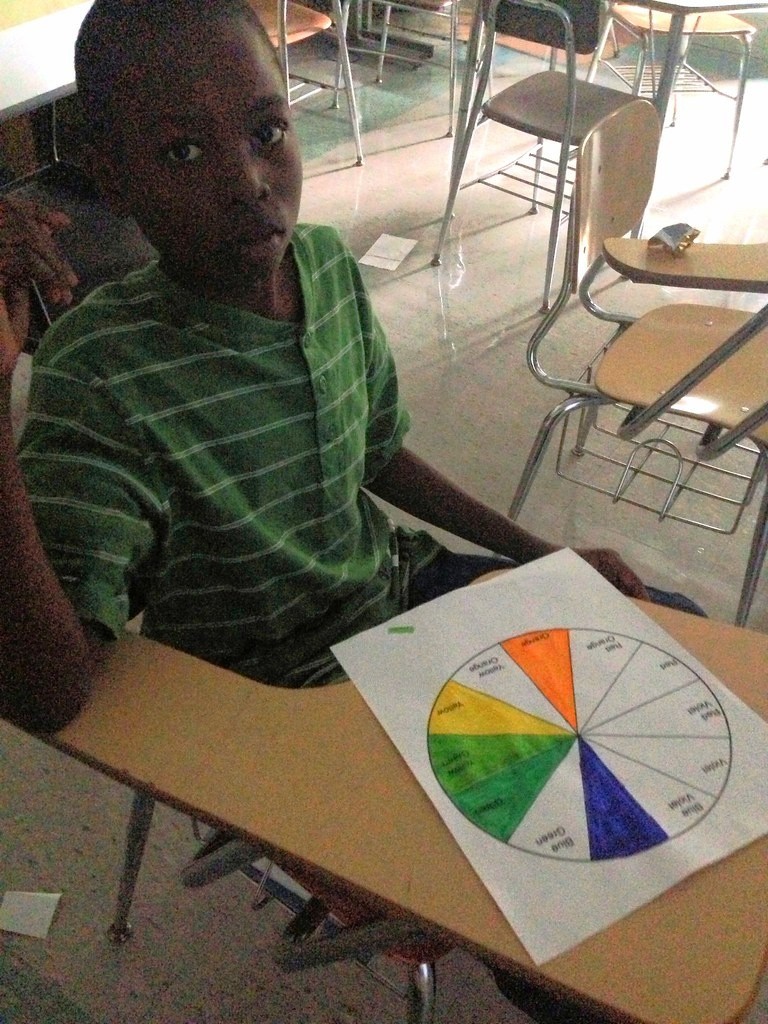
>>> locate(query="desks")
[0,569,768,1024]
[598,238,768,465]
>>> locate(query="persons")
[0,0,708,1024]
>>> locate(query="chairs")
[0,0,768,1024]
